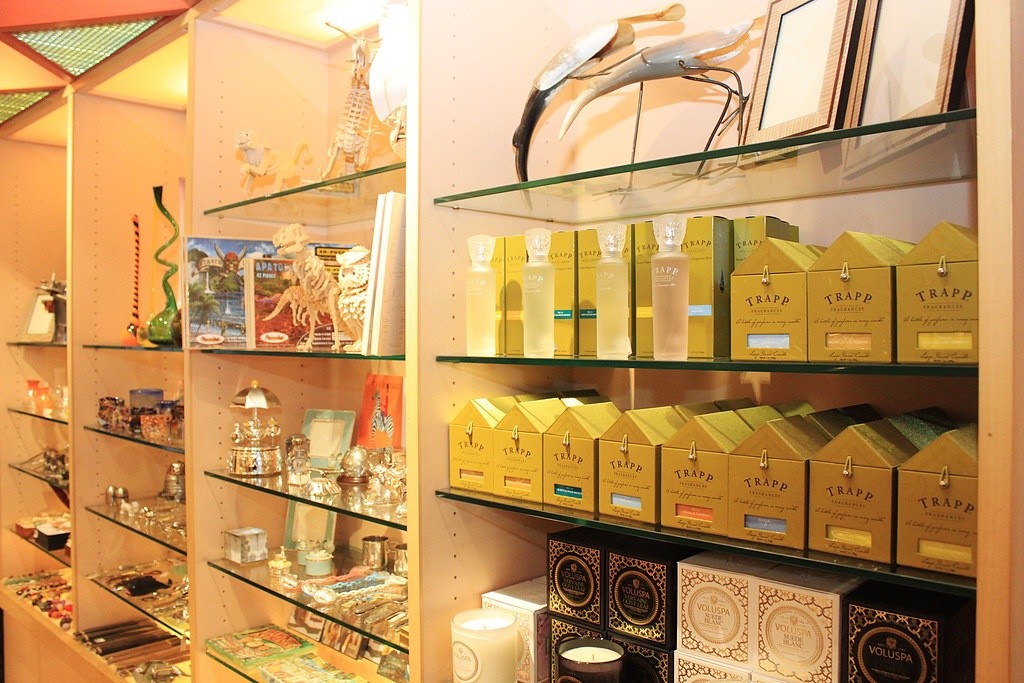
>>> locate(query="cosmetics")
[522,227,557,357]
[649,212,690,362]
[464,234,497,358]
[594,221,632,361]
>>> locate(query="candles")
[553,638,623,683]
[450,609,517,683]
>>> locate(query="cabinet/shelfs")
[435,107,980,683]
[6,341,72,570]
[84,346,193,642]
[198,160,408,683]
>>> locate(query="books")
[356,372,405,450]
[204,623,370,683]
[180,235,361,349]
[362,190,406,359]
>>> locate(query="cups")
[98,388,184,444]
[555,633,625,683]
[450,608,527,682]
[393,542,408,579]
[267,539,334,578]
[362,535,397,571]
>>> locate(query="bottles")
[594,221,629,360]
[524,228,555,358]
[650,216,690,361]
[467,234,497,359]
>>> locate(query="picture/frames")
[842,0,966,180]
[300,409,357,474]
[737,0,860,171]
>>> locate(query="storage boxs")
[449,389,980,683]
[470,215,979,365]
